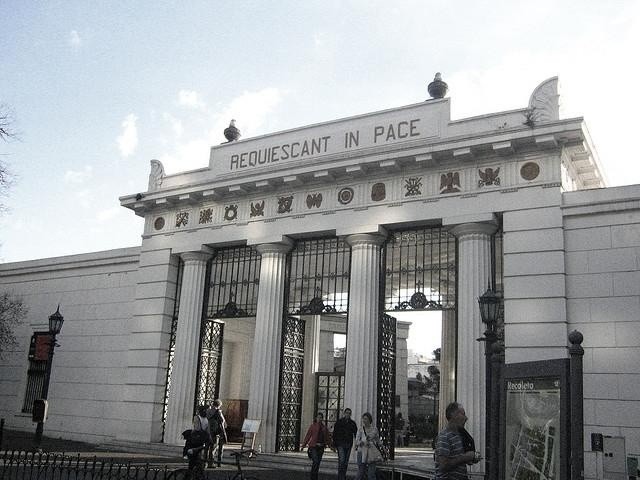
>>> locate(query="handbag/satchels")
[361,442,382,463]
[190,415,210,446]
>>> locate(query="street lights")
[33,303,64,446]
[423,375,436,450]
[476,277,501,480]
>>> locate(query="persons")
[191,407,214,468]
[434,402,481,480]
[208,399,227,467]
[332,408,358,480]
[395,411,406,447]
[354,413,388,480]
[300,412,336,480]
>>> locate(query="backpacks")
[208,409,223,435]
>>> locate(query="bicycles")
[165,446,259,480]
